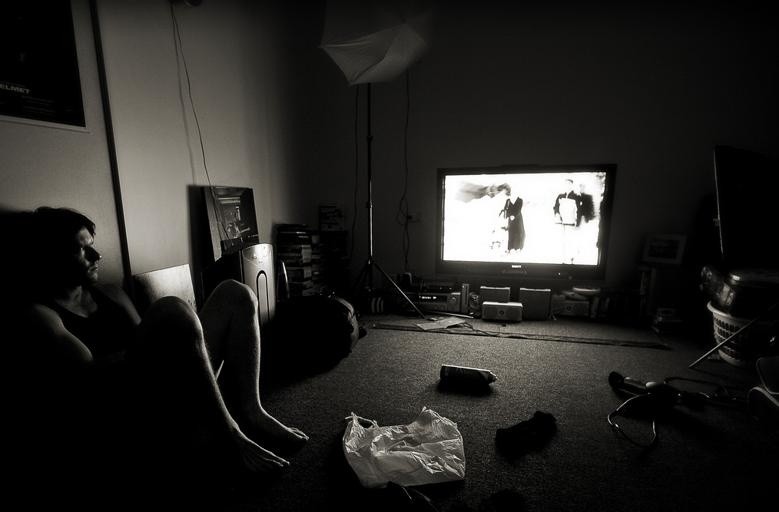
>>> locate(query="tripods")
[352,83,425,318]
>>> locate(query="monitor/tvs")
[436,164,618,277]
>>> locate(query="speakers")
[480,286,589,320]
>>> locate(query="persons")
[552,176,583,265]
[499,183,525,253]
[13,203,310,472]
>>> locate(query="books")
[270,221,325,298]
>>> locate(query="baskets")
[707,301,750,368]
